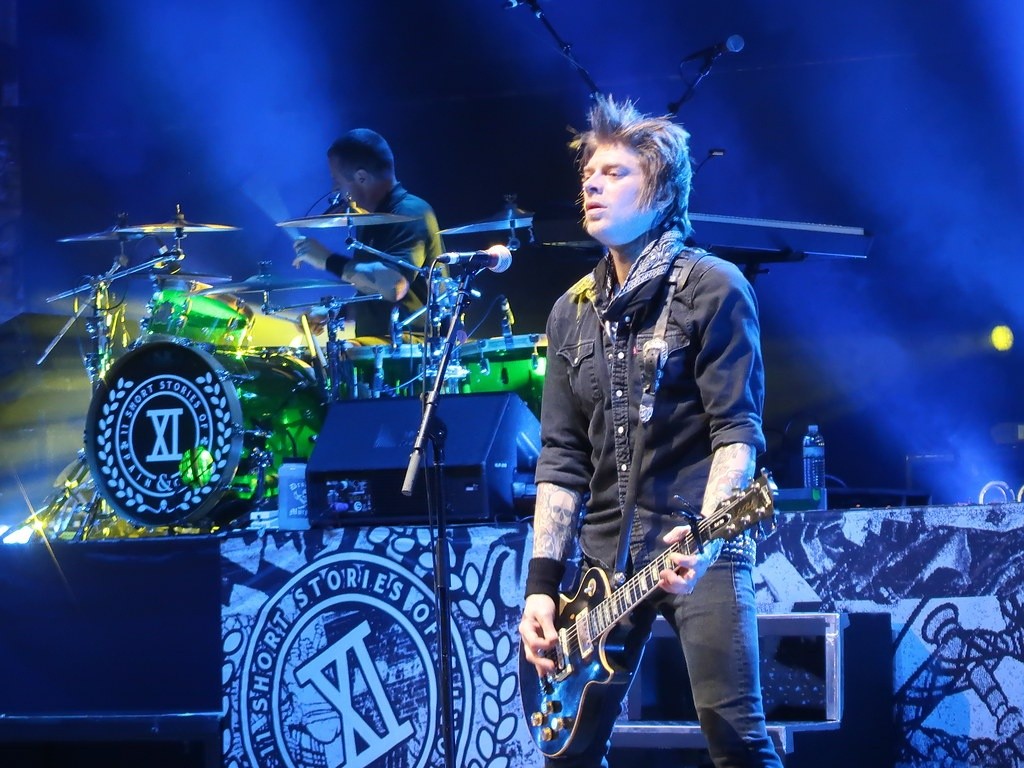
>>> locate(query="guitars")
[518,467,776,759]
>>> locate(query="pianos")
[542,208,877,288]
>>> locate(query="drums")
[83,332,320,529]
[448,332,548,422]
[253,346,313,367]
[139,280,256,351]
[331,340,442,401]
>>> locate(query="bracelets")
[524,557,565,600]
[324,254,349,278]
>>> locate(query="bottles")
[803,425,825,487]
[277,457,311,530]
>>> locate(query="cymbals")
[178,274,357,297]
[275,212,421,229]
[435,209,535,235]
[56,227,158,243]
[116,220,241,232]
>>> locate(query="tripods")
[0,205,185,543]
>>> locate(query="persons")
[287,128,452,346]
[518,94,787,768]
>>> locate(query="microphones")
[503,0,524,10]
[501,294,514,324]
[682,34,745,62]
[436,244,512,272]
[391,308,400,352]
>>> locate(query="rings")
[298,251,303,256]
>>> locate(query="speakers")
[306,391,542,526]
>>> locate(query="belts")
[723,533,757,567]
